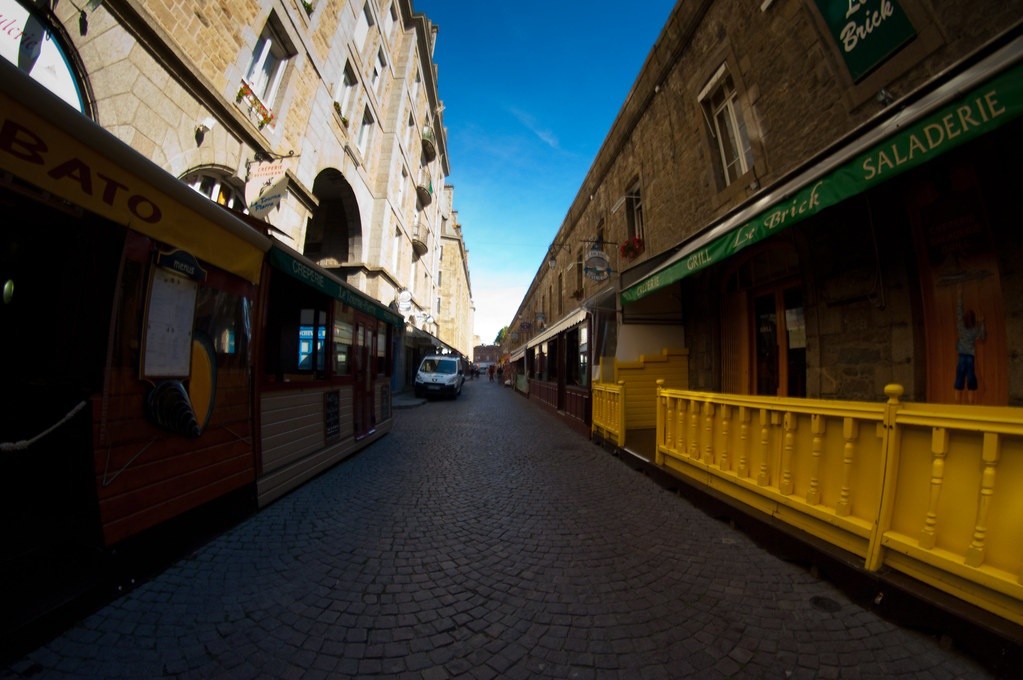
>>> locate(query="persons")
[497,366,503,384]
[475,369,480,378]
[488,365,495,383]
[954,283,986,404]
[470,369,474,380]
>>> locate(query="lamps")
[394,285,434,325]
[549,242,571,270]
[198,116,216,134]
[84,0,103,12]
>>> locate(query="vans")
[414,355,465,401]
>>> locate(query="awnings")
[407,323,464,356]
[509,344,527,363]
[617,19,1023,305]
[526,283,614,365]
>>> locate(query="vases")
[627,250,638,260]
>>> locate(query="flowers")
[236,81,276,133]
[617,235,649,259]
